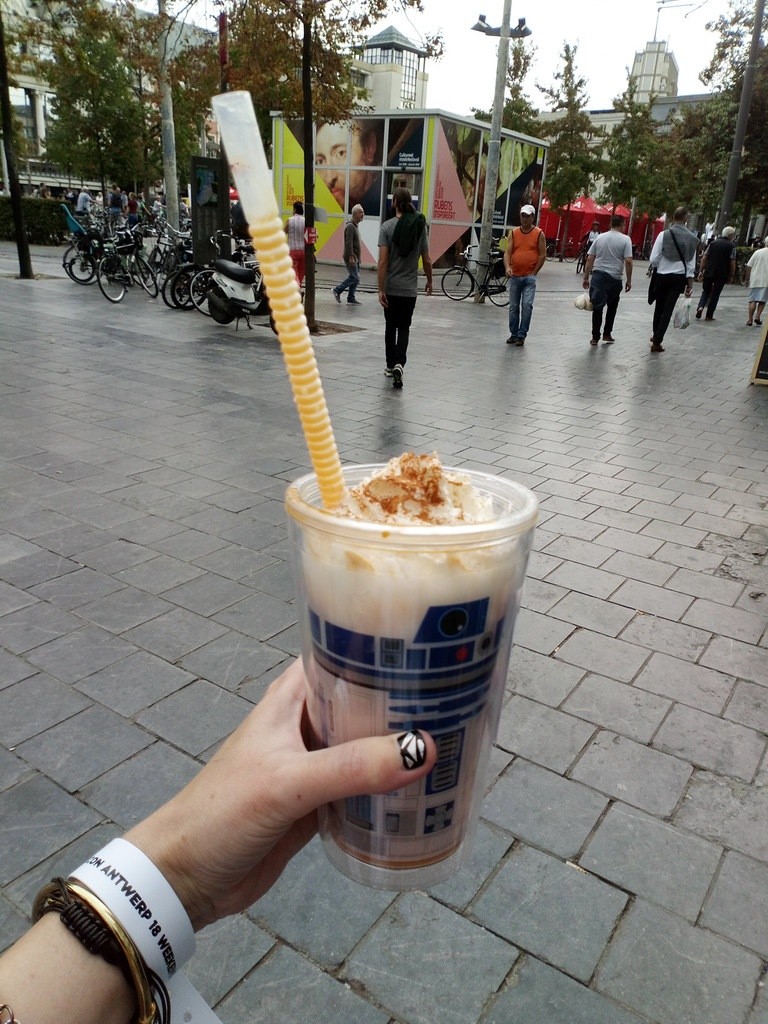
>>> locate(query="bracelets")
[0,837,224,1024]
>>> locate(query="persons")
[578,221,602,261]
[315,117,385,217]
[21,182,252,242]
[582,214,633,346]
[744,236,768,326]
[503,204,546,347]
[377,186,433,389]
[696,230,765,250]
[646,206,695,352]
[283,201,306,304]
[0,654,437,1024]
[696,226,737,321]
[331,204,364,306]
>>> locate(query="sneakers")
[507,336,516,343]
[346,299,362,306]
[383,366,393,377]
[602,336,615,343]
[392,364,403,389]
[651,344,665,352]
[331,289,341,303]
[590,339,598,345]
[515,337,525,346]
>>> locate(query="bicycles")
[57,201,281,337]
[441,242,515,307]
[576,243,587,274]
[546,237,580,263]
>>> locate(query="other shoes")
[705,317,715,320]
[754,319,762,325]
[696,307,703,318]
[746,320,752,325]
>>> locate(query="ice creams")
[296,450,508,871]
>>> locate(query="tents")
[538,181,665,259]
[229,186,239,200]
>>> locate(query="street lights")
[470,4,533,310]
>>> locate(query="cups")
[281,461,540,892]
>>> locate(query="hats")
[520,205,535,215]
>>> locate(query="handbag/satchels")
[574,288,593,311]
[674,296,692,329]
[388,210,426,259]
